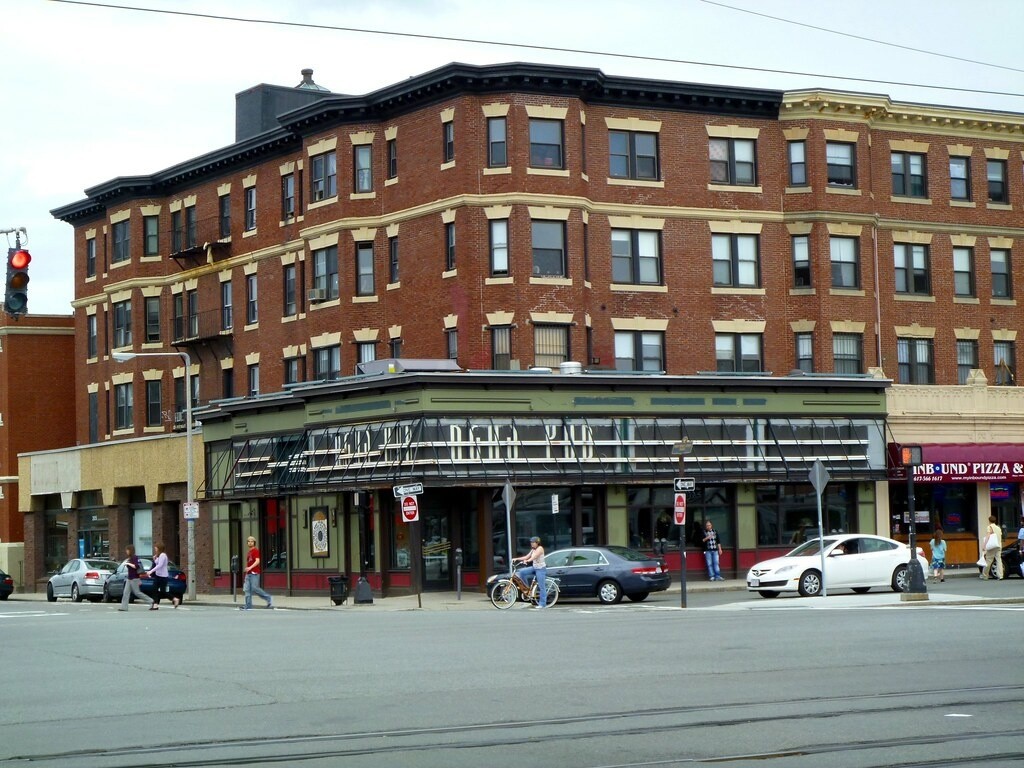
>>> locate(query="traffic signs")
[393,482,424,498]
[673,478,696,491]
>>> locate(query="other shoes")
[980,574,988,580]
[267,598,272,607]
[716,576,724,581]
[710,576,716,581]
[933,577,938,584]
[118,608,128,612]
[940,578,945,582]
[173,598,180,609]
[149,607,158,610]
[517,586,526,589]
[536,605,544,609]
[240,605,252,610]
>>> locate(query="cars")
[485,545,672,604]
[0,568,14,600]
[747,533,930,599]
[47,558,120,604]
[103,554,188,606]
[979,539,1024,580]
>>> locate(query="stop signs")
[400,495,419,523]
[675,493,686,525]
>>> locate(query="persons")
[239,536,272,611]
[692,522,703,548]
[789,525,807,546]
[928,530,947,584]
[511,536,547,609]
[700,520,726,581]
[147,541,181,610]
[354,576,373,604]
[979,516,1004,581]
[118,545,153,611]
[1017,517,1024,555]
[836,543,849,556]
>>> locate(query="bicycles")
[490,560,561,610]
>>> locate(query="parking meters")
[231,555,239,602]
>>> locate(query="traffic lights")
[4,248,32,316]
[901,445,923,467]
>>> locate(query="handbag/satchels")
[150,561,156,578]
[984,526,1000,550]
[136,560,146,574]
[976,552,987,568]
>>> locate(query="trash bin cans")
[328,575,350,606]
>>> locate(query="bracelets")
[1019,550,1023,553]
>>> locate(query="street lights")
[111,353,196,601]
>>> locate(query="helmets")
[530,537,540,541]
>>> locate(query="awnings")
[888,441,1024,482]
